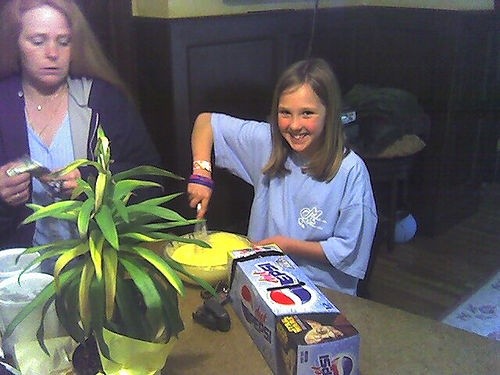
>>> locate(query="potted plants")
[1,123,218,375]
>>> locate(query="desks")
[142,241,500,375]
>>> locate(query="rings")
[16,193,20,200]
[59,180,63,192]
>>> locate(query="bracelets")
[188,174,215,189]
[192,161,212,172]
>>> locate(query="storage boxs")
[226,242,360,375]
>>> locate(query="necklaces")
[24,79,67,110]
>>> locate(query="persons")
[188,57,378,296]
[0,0,175,278]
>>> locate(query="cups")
[0,248,56,355]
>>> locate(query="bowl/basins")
[163,229,253,287]
